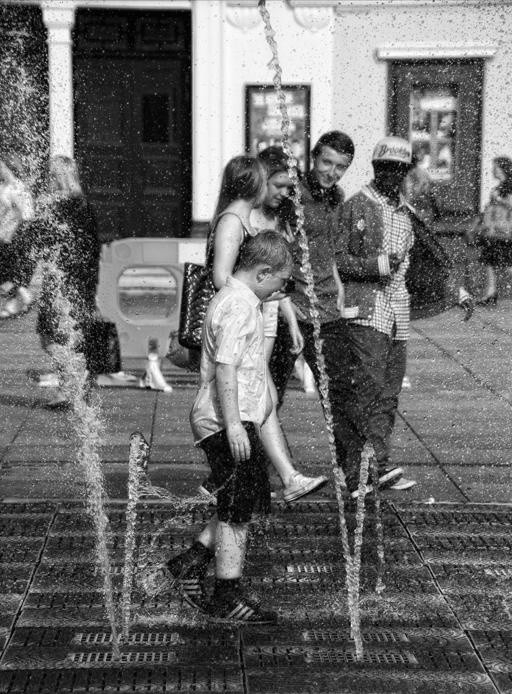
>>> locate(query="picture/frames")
[244,83,312,177]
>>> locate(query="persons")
[193,157,329,507]
[0,156,36,319]
[161,230,295,625]
[277,131,405,500]
[474,156,512,305]
[30,156,101,412]
[337,137,476,492]
[257,147,323,406]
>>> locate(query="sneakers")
[391,476,414,491]
[164,554,211,614]
[350,466,403,499]
[209,594,278,625]
[282,474,326,504]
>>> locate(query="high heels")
[478,292,497,307]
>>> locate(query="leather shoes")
[42,400,70,411]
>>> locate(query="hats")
[372,136,412,166]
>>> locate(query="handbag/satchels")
[178,263,218,349]
[78,320,119,373]
[480,203,511,242]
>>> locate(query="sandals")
[0,302,27,321]
